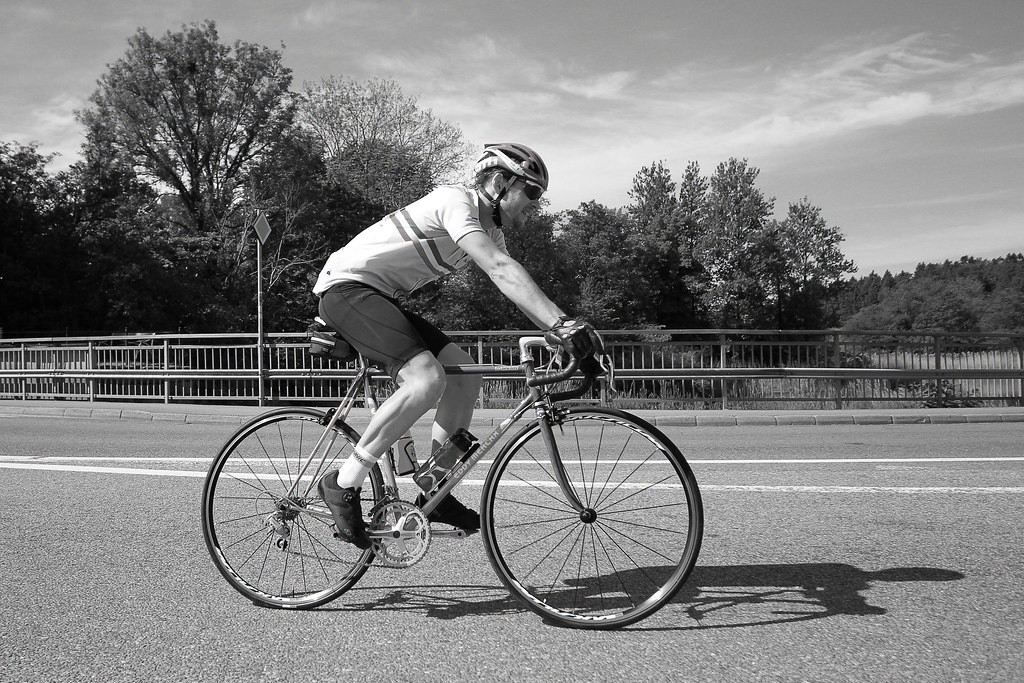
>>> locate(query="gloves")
[542,316,607,362]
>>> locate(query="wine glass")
[412,428,478,493]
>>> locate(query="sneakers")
[315,470,374,549]
[413,489,485,529]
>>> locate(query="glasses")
[516,180,543,202]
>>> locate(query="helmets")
[472,141,552,192]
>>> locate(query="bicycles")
[200,328,702,630]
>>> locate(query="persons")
[313,143,604,549]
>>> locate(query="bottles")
[390,428,419,475]
